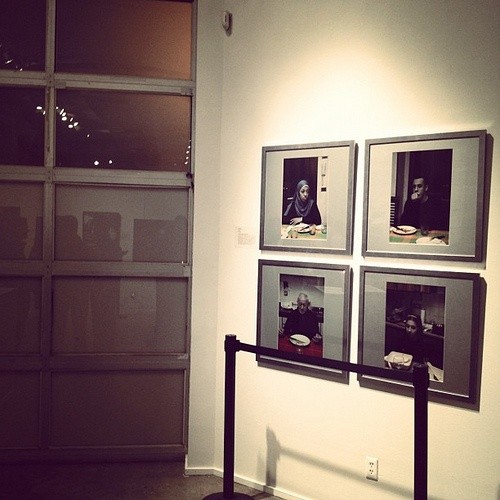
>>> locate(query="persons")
[398,173,445,232]
[388,312,431,367]
[278,292,323,344]
[282,180,322,227]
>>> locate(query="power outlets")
[363,455,380,481]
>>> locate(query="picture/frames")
[255,260,351,379]
[259,140,356,255]
[356,264,482,405]
[361,128,487,261]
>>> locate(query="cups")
[421,225,429,235]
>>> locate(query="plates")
[294,224,311,232]
[289,334,310,346]
[393,225,417,235]
[416,237,447,245]
